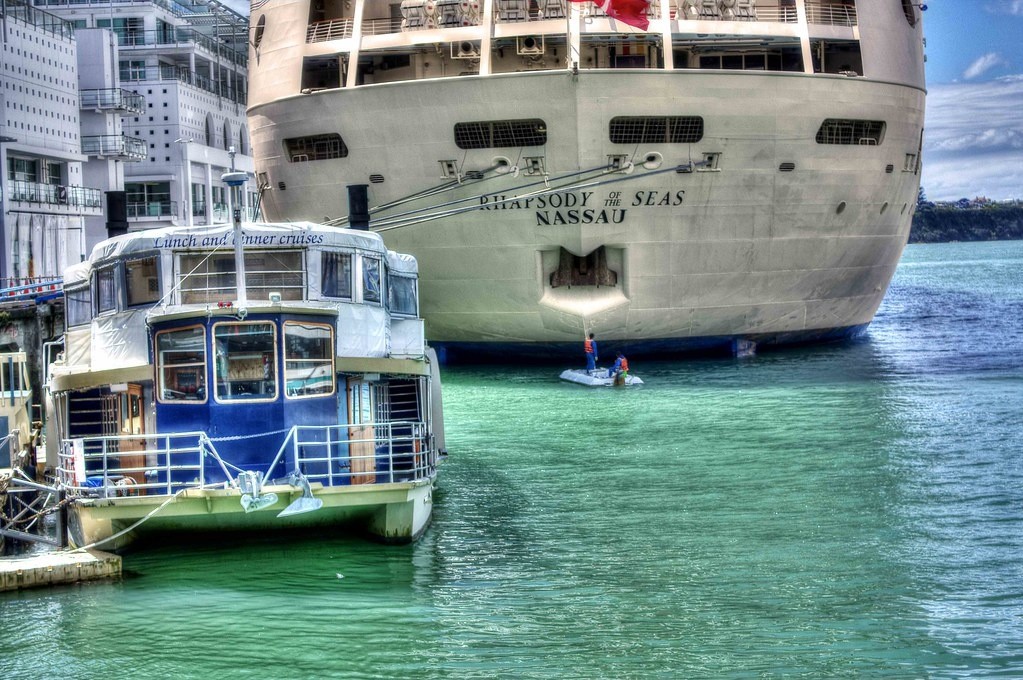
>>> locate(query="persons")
[584,333,599,375]
[609,351,629,377]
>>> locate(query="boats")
[1,350,40,552]
[560,366,643,386]
[42,173,444,551]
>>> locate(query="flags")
[566,0,650,31]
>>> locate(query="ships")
[246,0,931,365]
